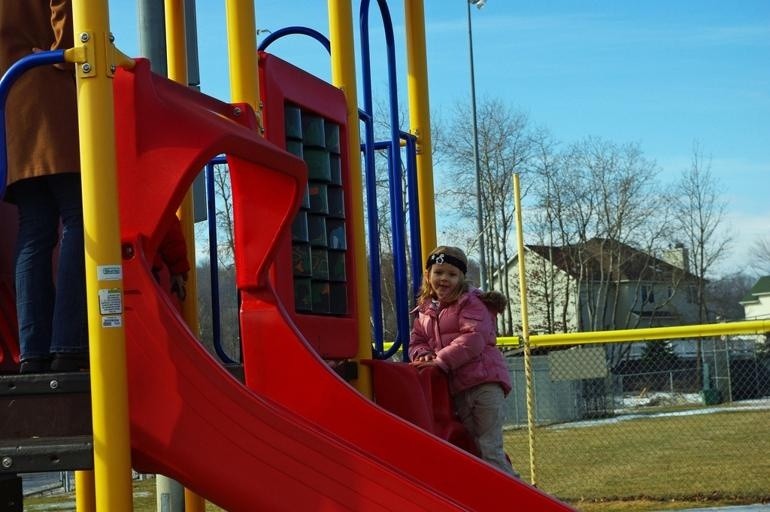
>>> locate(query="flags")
[468,0,488,10]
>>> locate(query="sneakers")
[20,354,81,373]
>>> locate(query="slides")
[120,257,581,512]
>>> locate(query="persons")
[409,245,532,479]
[0,0,90,375]
